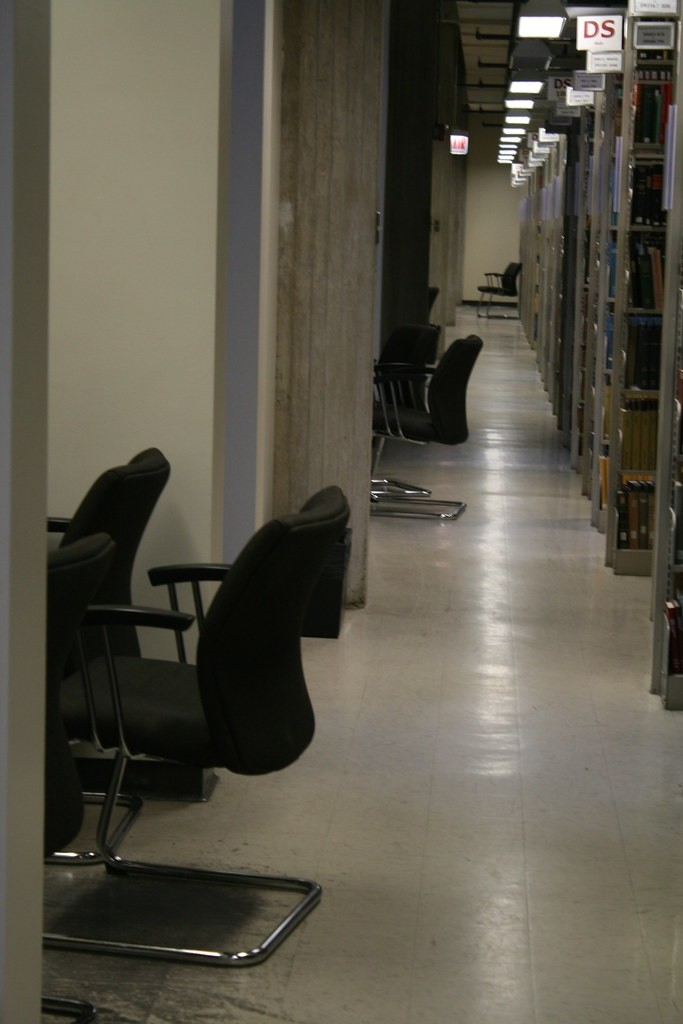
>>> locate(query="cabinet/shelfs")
[521,0,683,710]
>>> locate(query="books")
[535,15,683,677]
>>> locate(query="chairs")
[43,446,170,865]
[370,286,483,520]
[42,487,352,968]
[42,532,119,1024]
[476,262,522,319]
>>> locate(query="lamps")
[496,0,570,165]
